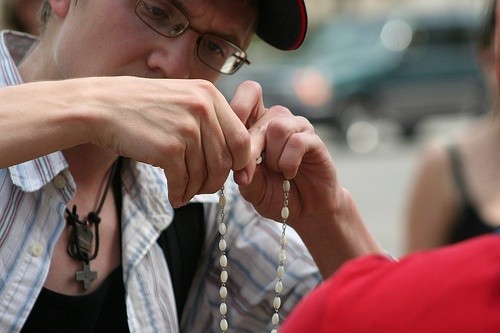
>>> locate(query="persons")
[0,0,500,333]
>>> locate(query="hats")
[255,0,308,50]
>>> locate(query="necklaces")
[66,161,118,291]
[219,156,291,333]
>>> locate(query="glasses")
[135,0,251,74]
